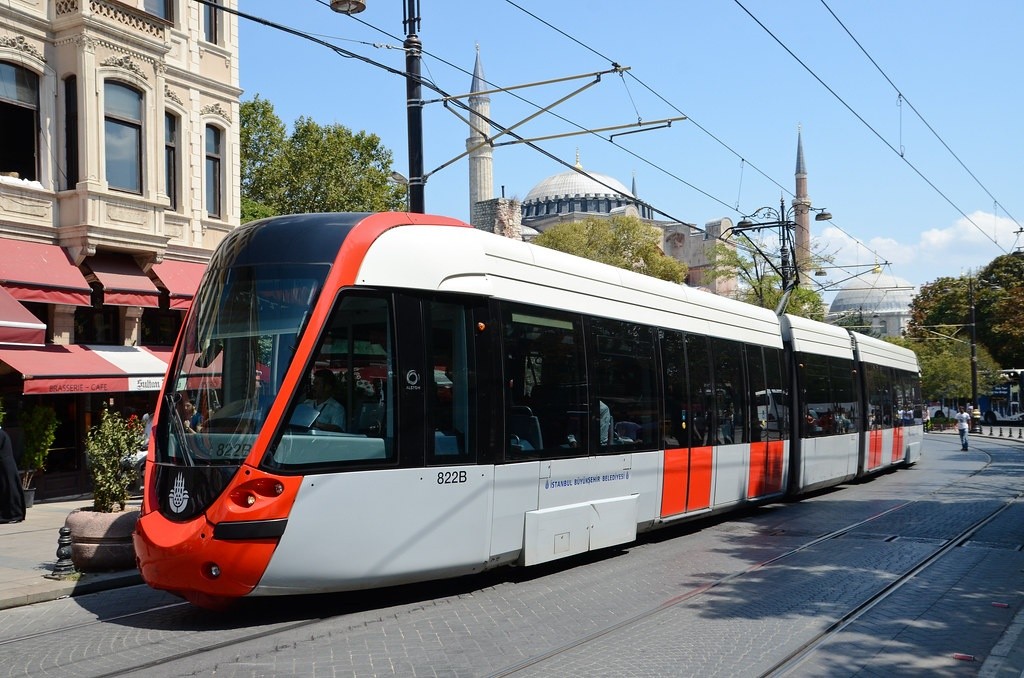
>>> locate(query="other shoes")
[961,448,968,451]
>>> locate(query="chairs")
[510,404,543,452]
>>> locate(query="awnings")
[0,284,47,346]
[0,343,269,394]
[0,238,208,311]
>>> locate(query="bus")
[132,211,926,624]
[901,366,1024,427]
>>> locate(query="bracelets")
[186,427,190,430]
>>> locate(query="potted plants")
[18,402,61,508]
[65,400,150,572]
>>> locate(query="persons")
[869,408,889,430]
[141,407,157,443]
[703,403,734,447]
[549,401,610,456]
[895,406,914,426]
[306,370,346,433]
[183,398,221,435]
[806,409,851,436]
[954,407,971,451]
[0,426,26,524]
[922,405,930,434]
